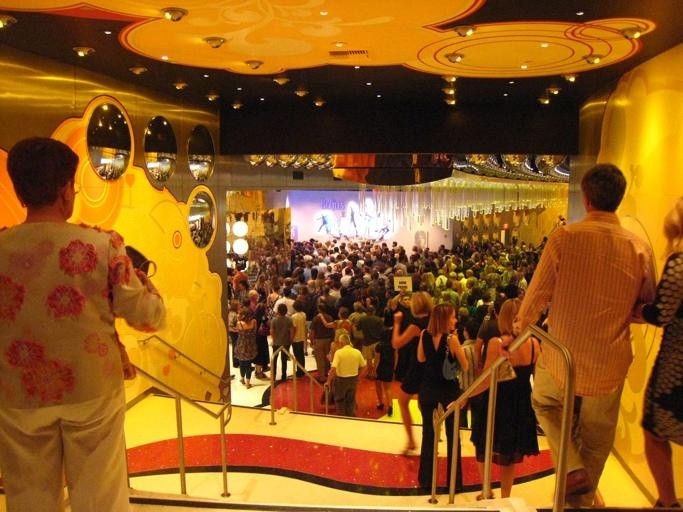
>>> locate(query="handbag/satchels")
[257,322,271,337]
[496,336,518,383]
[441,333,461,380]
[320,390,334,406]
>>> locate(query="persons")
[511,162,658,508]
[633,196,683,508]
[0,136,167,511]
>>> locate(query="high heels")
[375,404,385,408]
[387,406,392,416]
[403,442,416,457]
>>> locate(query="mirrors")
[188,191,216,249]
[143,115,178,182]
[186,124,216,183]
[86,101,131,182]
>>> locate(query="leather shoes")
[565,469,590,501]
[240,380,253,389]
[255,365,270,378]
